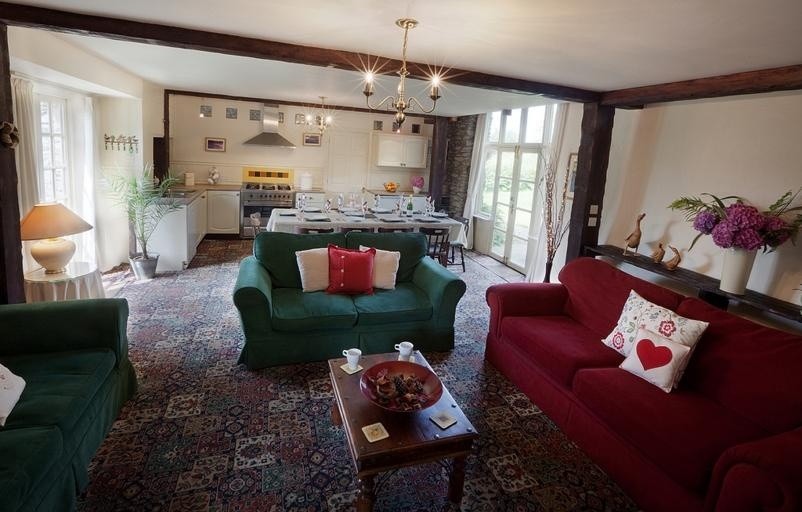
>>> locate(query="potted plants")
[100,163,185,281]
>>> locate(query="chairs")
[419,226,446,267]
[441,217,471,272]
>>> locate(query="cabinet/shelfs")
[377,134,427,169]
[135,191,207,272]
[208,191,241,234]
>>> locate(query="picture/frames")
[563,152,579,199]
[303,132,322,147]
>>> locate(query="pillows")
[294,247,329,293]
[328,243,376,296]
[359,246,400,290]
[619,328,691,394]
[600,289,709,390]
[1,365,26,427]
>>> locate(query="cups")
[343,348,361,370]
[394,341,414,358]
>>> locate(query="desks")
[24,261,107,304]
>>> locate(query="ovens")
[241,205,293,240]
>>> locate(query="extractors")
[240,102,297,150]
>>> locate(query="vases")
[719,248,758,295]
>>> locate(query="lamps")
[362,19,442,127]
[20,202,93,274]
[305,97,333,131]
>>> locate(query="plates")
[343,211,364,216]
[430,410,457,429]
[339,208,358,212]
[429,212,448,218]
[380,216,402,222]
[303,213,329,221]
[415,216,439,222]
[340,362,363,375]
[279,210,297,216]
[359,360,443,413]
[397,355,415,363]
[304,207,322,212]
[362,423,389,443]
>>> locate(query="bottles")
[407,198,413,217]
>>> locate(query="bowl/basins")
[383,182,400,193]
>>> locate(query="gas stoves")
[241,181,294,206]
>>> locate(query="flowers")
[667,191,802,255]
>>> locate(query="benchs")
[233,231,466,371]
[485,258,792,511]
[1,297,135,512]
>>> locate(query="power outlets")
[587,218,599,227]
[590,205,599,214]
